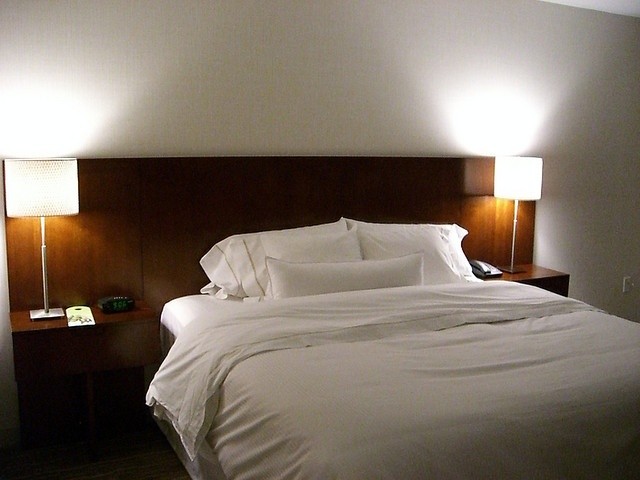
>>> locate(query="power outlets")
[622,275,633,293]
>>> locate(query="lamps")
[3,156,84,321]
[491,154,545,275]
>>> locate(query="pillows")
[230,222,364,302]
[198,217,346,301]
[264,248,425,300]
[346,218,470,284]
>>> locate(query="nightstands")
[487,263,570,298]
[8,298,162,450]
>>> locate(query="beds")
[156,280,639,479]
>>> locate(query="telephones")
[468,260,503,280]
